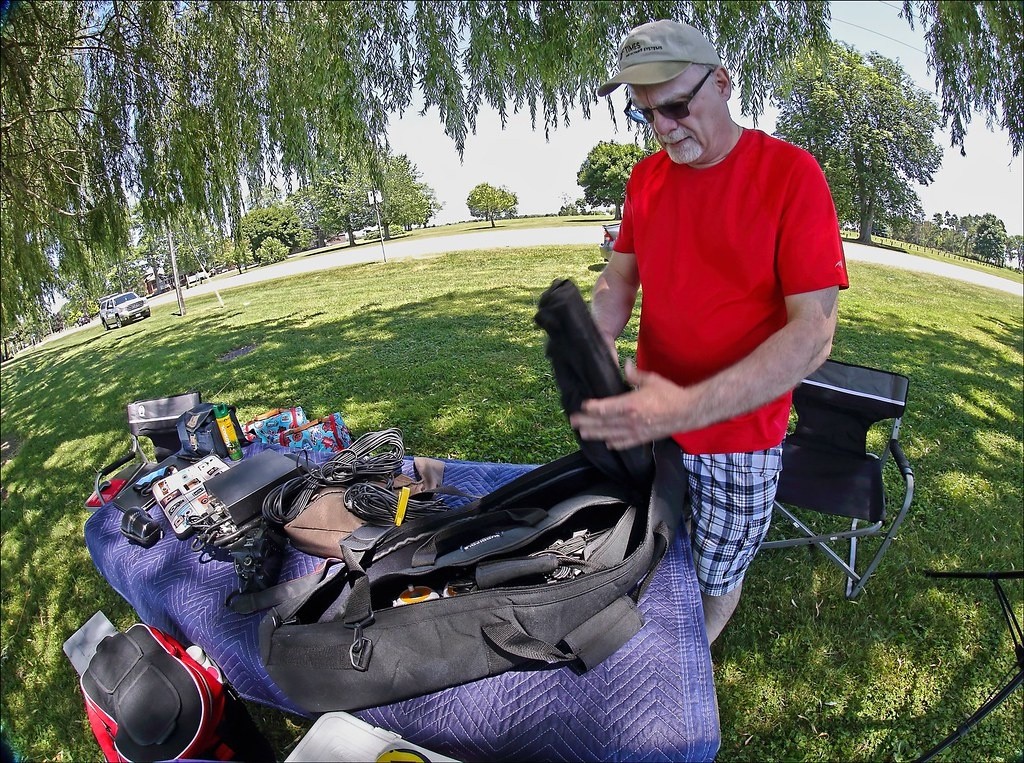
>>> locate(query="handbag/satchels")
[176,403,244,458]
[272,412,352,452]
[244,406,308,445]
[258,434,687,713]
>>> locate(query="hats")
[597,20,720,97]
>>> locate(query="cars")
[78,315,90,326]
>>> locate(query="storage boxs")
[284,712,461,763]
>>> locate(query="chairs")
[95,390,201,507]
[758,360,914,600]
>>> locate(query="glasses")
[624,67,714,124]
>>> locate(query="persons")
[570,19,850,650]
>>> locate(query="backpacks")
[80,623,226,763]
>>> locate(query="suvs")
[97,291,151,331]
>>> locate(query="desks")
[82,454,723,763]
